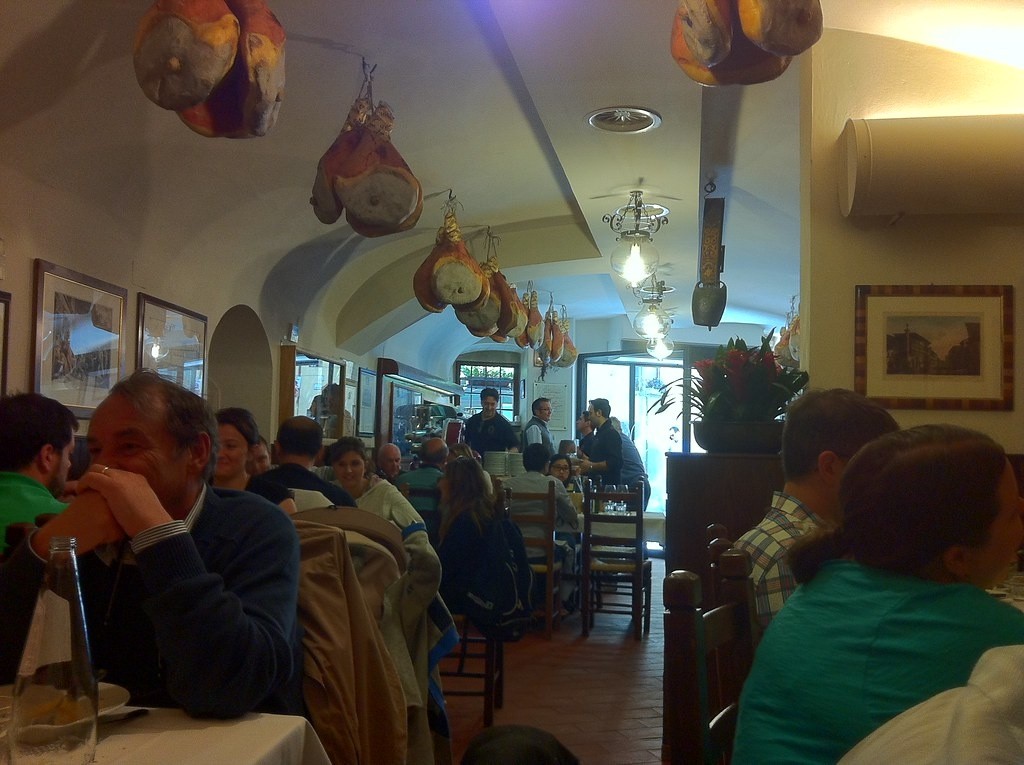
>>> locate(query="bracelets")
[589,462,592,470]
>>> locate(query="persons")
[437,456,548,643]
[493,443,580,626]
[209,406,423,528]
[558,399,650,513]
[375,442,406,482]
[0,393,80,560]
[463,388,523,467]
[549,453,572,489]
[729,387,899,684]
[394,437,448,550]
[0,368,302,721]
[520,398,554,463]
[309,384,354,439]
[730,424,1024,765]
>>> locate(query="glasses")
[537,407,553,412]
[552,466,570,472]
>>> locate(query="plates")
[17,682,130,745]
[483,452,527,477]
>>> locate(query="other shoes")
[558,600,576,620]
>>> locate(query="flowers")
[646,326,810,420]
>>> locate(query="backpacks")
[470,519,533,643]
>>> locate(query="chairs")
[298,478,561,731]
[661,523,751,765]
[575,479,652,641]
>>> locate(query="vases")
[687,419,786,454]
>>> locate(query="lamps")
[601,191,675,362]
[385,359,465,399]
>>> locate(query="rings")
[102,467,110,473]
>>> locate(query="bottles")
[8,537,98,765]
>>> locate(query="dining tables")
[5,707,333,765]
[563,510,666,550]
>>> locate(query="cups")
[603,502,627,516]
[604,485,629,493]
[0,696,12,765]
[1011,576,1024,609]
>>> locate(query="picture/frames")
[854,284,1015,412]
[136,292,207,400]
[356,367,377,437]
[30,257,129,419]
